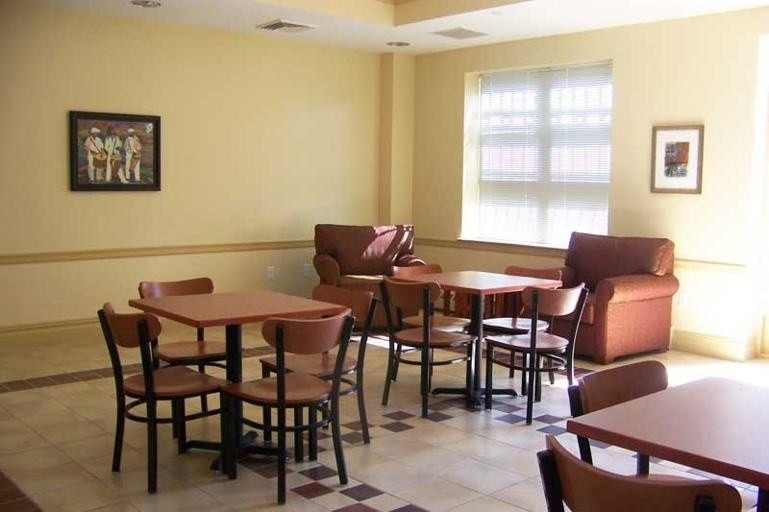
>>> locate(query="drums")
[130,155,139,170]
[110,156,122,175]
[93,154,106,169]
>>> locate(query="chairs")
[220,305,356,504]
[381,272,479,418]
[99,303,233,495]
[483,265,563,394]
[311,222,429,332]
[494,232,680,364]
[566,358,670,467]
[258,284,378,445]
[137,277,246,436]
[391,262,473,392]
[536,431,744,512]
[483,280,590,423]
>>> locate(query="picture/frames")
[649,124,705,196]
[68,110,162,190]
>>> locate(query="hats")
[91,128,100,133]
[128,129,135,133]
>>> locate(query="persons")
[83,125,143,184]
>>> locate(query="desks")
[567,374,769,512]
[127,287,349,479]
[384,270,563,411]
